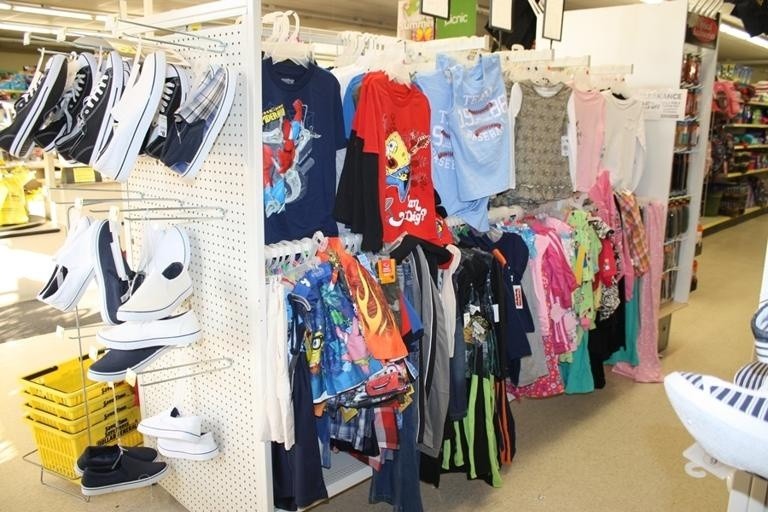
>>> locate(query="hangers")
[262,10,637,100]
[264,193,520,282]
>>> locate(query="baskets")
[21,347,143,485]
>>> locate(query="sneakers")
[73,406,219,497]
[37,215,201,384]
[0,51,237,183]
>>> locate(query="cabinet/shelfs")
[716,97,768,178]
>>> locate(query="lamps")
[718,21,768,50]
